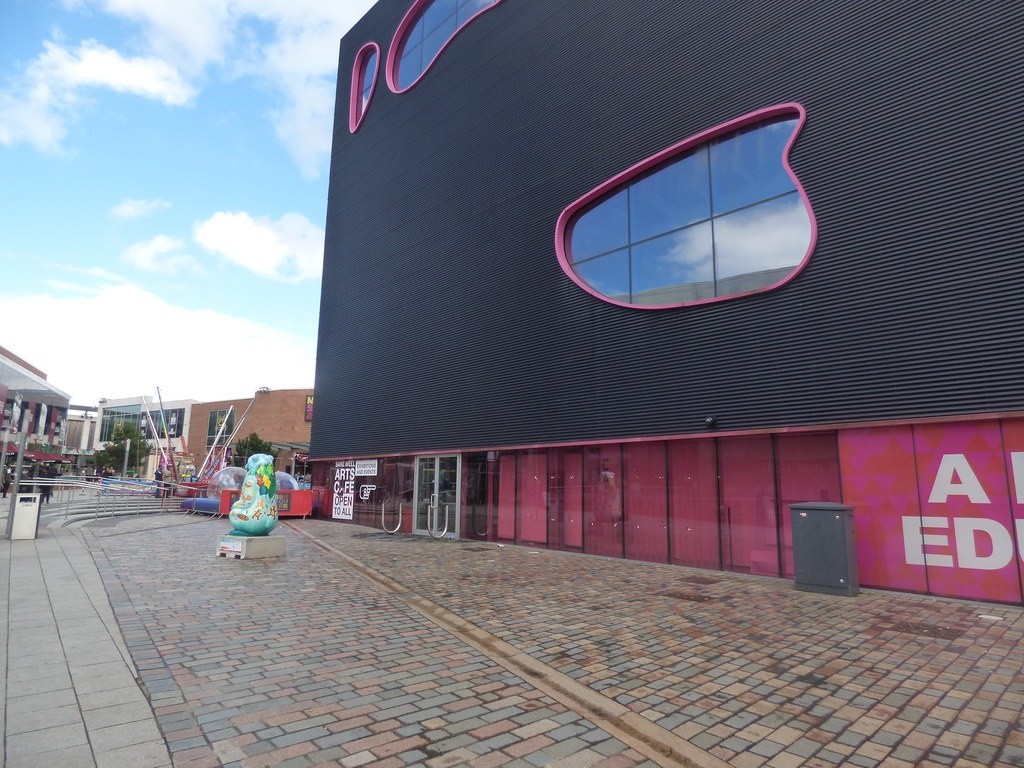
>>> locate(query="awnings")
[0,442,70,462]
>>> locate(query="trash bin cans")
[5,493,43,540]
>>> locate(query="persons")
[2,460,64,504]
[163,466,173,498]
[85,463,115,482]
[155,465,163,498]
